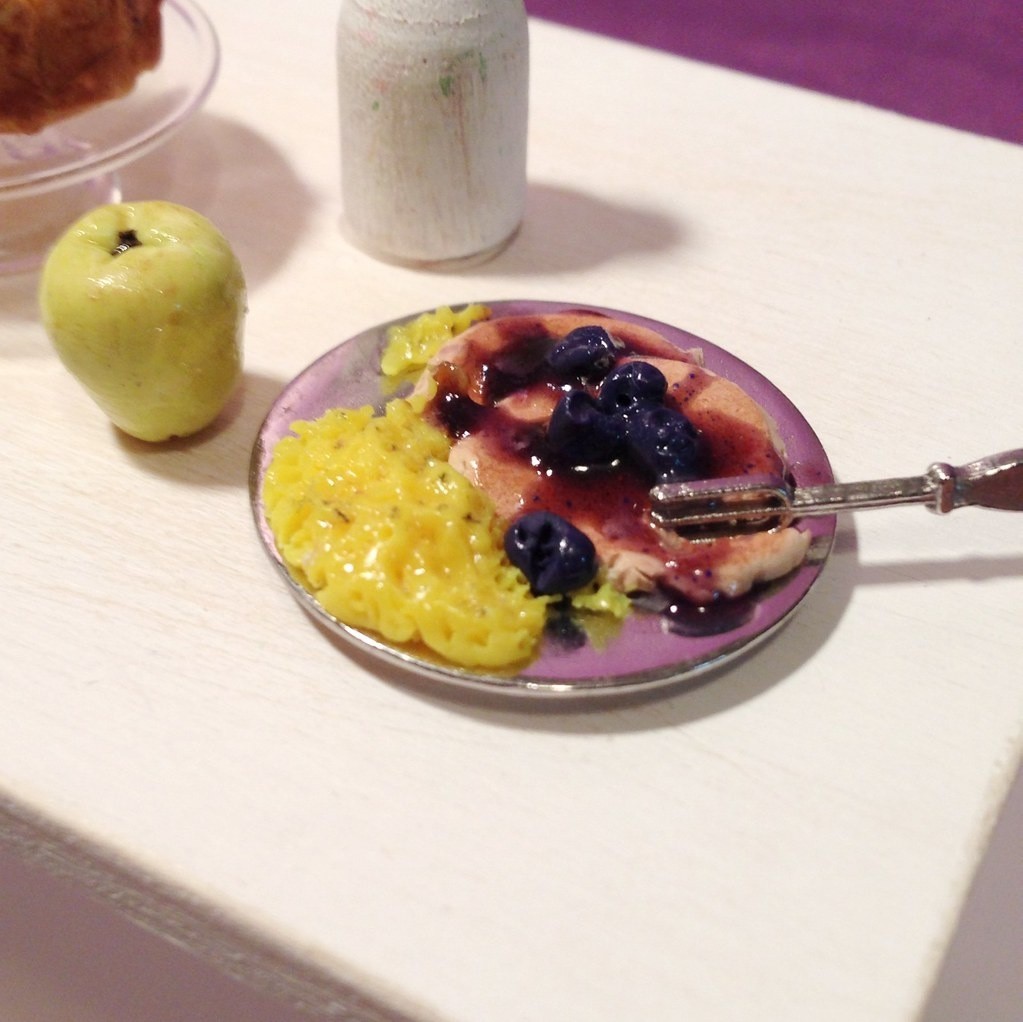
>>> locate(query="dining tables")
[1,0,1022,1022]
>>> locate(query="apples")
[38,200,245,441]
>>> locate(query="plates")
[1,0,220,201]
[249,299,835,699]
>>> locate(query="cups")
[334,0,529,261]
